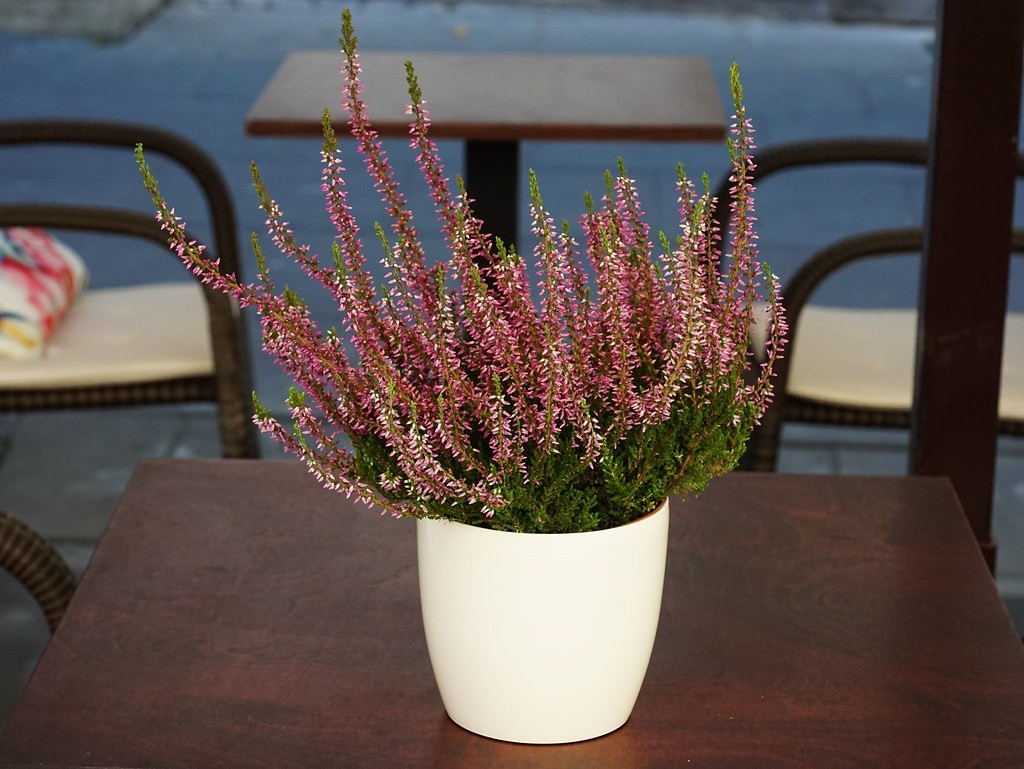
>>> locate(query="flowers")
[134,8,791,532]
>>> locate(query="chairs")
[699,136,1024,472]
[0,119,262,457]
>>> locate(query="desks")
[246,49,726,290]
[0,457,1024,769]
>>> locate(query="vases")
[417,492,670,745]
